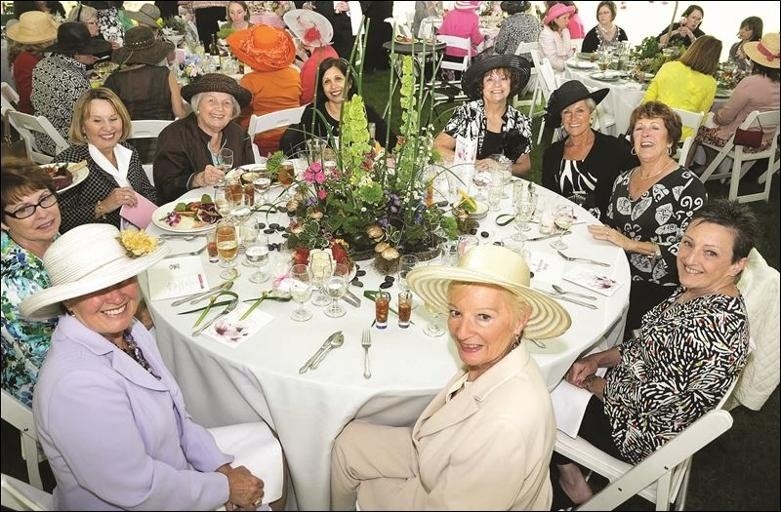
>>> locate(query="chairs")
[0,17,780,510]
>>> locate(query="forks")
[557,250,613,267]
[361,326,373,378]
[160,234,198,241]
[167,244,208,259]
[191,300,240,335]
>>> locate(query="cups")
[374,295,391,328]
[397,291,414,329]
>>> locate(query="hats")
[454,1,575,24]
[19,222,175,323]
[405,245,571,340]
[5,4,169,66]
[545,79,610,130]
[460,52,532,101]
[743,32,780,68]
[179,8,333,109]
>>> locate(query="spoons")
[311,335,343,369]
[192,281,234,311]
[550,285,599,301]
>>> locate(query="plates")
[393,35,447,47]
[151,197,223,232]
[571,51,655,84]
[712,89,732,99]
[36,162,90,195]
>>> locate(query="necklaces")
[638,160,674,180]
[680,280,738,303]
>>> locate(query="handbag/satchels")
[732,112,764,148]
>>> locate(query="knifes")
[537,287,598,309]
[299,328,344,374]
[172,282,227,308]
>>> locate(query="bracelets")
[648,241,658,259]
[585,375,603,391]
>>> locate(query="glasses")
[3,191,59,220]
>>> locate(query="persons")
[332,244,572,512]
[589,102,707,343]
[552,198,761,511]
[1,0,780,235]
[0,155,156,490]
[21,221,288,510]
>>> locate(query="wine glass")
[207,138,346,322]
[395,253,421,308]
[472,155,575,250]
[423,303,447,338]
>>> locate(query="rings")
[254,499,263,506]
[606,231,609,236]
